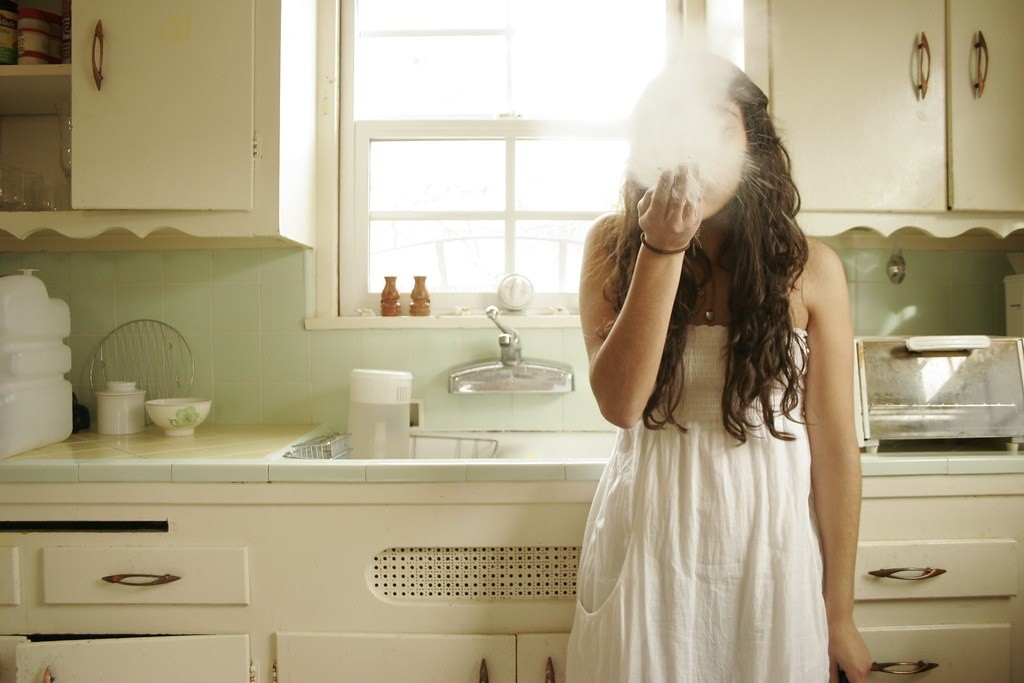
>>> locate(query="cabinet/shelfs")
[0,476,1024,683]
[703,0,1023,240]
[0,0,316,264]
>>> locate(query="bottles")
[95,381,145,435]
[410,276,430,316]
[382,277,400,316]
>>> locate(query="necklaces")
[703,296,716,322]
[640,232,689,254]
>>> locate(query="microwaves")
[855,334,1024,453]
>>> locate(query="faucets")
[484,304,524,366]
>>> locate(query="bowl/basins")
[146,398,212,435]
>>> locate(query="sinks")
[282,418,619,470]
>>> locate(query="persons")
[565,54,874,683]
